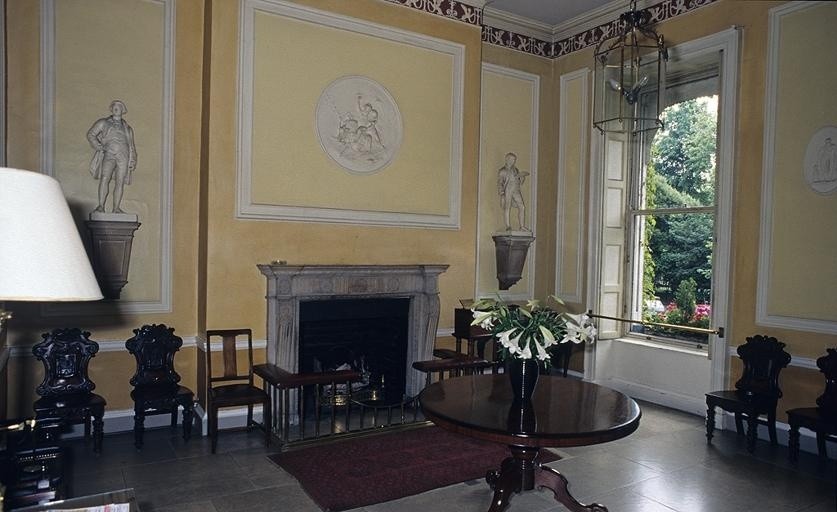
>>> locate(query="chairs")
[705,333,791,454]
[124,315,194,442]
[784,344,836,459]
[204,327,273,454]
[31,323,109,454]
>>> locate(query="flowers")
[470,289,614,373]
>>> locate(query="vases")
[505,358,541,399]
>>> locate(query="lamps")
[0,168,107,435]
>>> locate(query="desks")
[417,373,643,511]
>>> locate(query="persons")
[811,162,820,182]
[496,151,531,234]
[86,98,140,214]
[819,137,836,183]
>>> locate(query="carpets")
[263,423,569,512]
